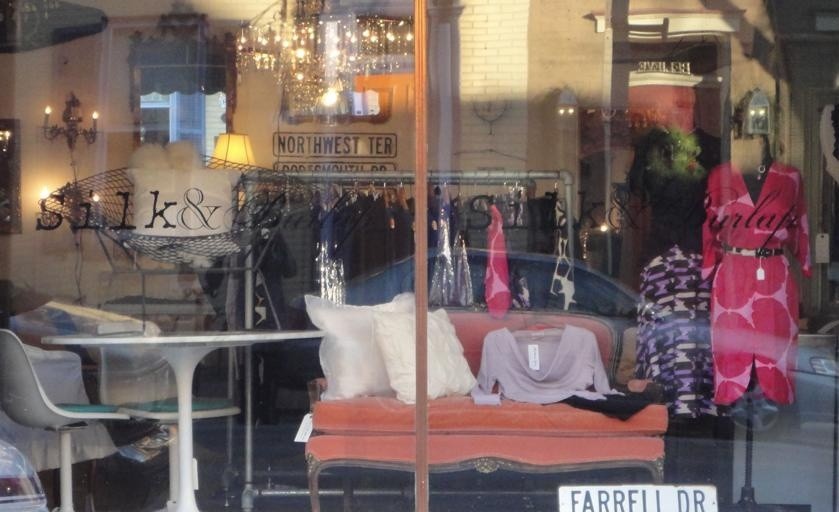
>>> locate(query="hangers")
[242,172,567,230]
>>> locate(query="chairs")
[1,328,241,512]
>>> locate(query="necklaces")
[754,153,771,173]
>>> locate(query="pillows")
[301,292,477,402]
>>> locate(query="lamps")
[42,94,101,148]
[209,131,256,171]
[736,86,773,140]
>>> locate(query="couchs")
[305,309,671,512]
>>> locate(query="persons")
[698,133,814,407]
[615,129,678,319]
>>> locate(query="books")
[8,298,145,338]
